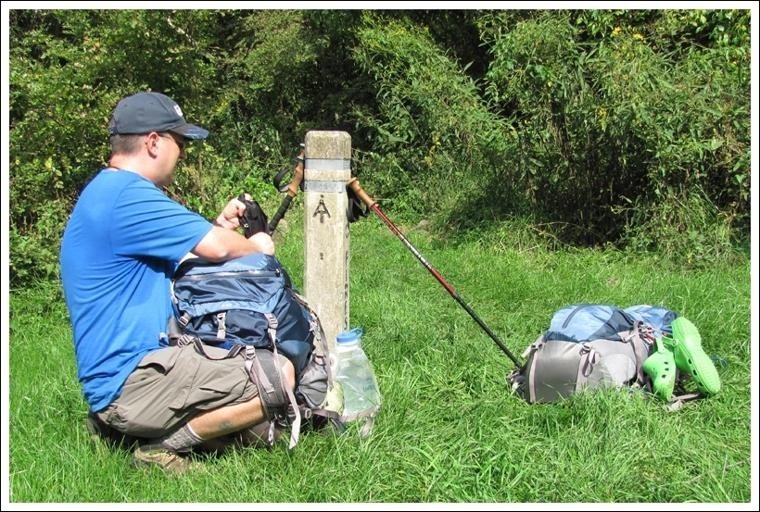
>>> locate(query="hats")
[106,92,210,141]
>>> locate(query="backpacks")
[506,303,677,405]
[170,254,334,451]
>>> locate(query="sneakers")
[131,441,208,477]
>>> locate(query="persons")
[60,89,335,476]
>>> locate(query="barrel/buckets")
[331,326,381,416]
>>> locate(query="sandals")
[661,317,721,397]
[641,337,676,402]
